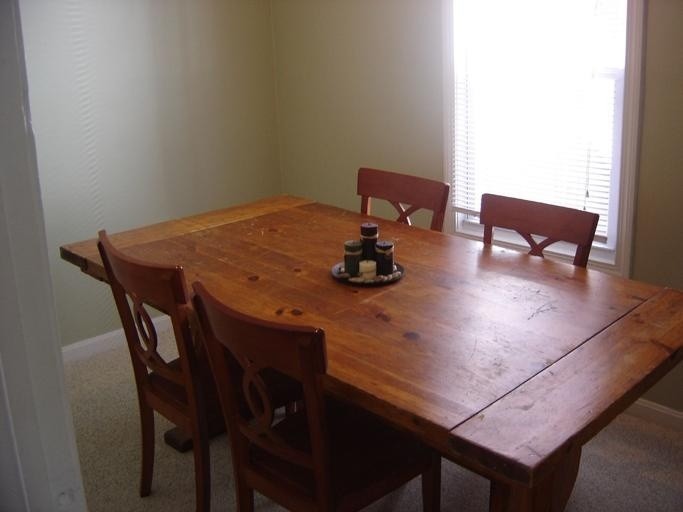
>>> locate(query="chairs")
[354,166,600,270]
[99,228,304,511]
[192,283,439,512]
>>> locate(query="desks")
[58,195,683,512]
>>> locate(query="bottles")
[342,223,394,278]
